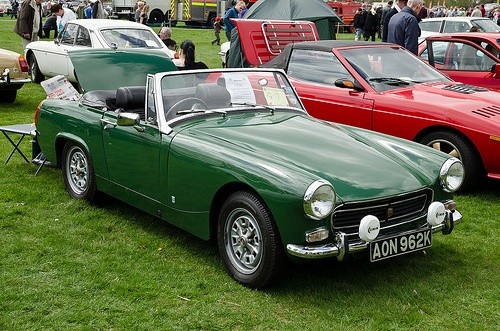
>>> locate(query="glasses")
[240,7,244,10]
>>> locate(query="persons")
[11,0,254,84]
[351,0,500,60]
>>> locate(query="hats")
[215,17,221,21]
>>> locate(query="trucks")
[324,0,391,34]
[0,0,236,28]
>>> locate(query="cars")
[0,47,33,103]
[483,3,497,17]
[418,16,500,59]
[416,30,500,95]
[23,18,179,97]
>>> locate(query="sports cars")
[207,17,500,198]
[30,65,468,289]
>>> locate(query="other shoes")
[211,41,213,45]
[217,43,220,45]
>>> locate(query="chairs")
[195,83,231,109]
[116,85,157,119]
[482,45,497,68]
[460,44,480,69]
[0,123,47,176]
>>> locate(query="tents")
[227,0,346,69]
